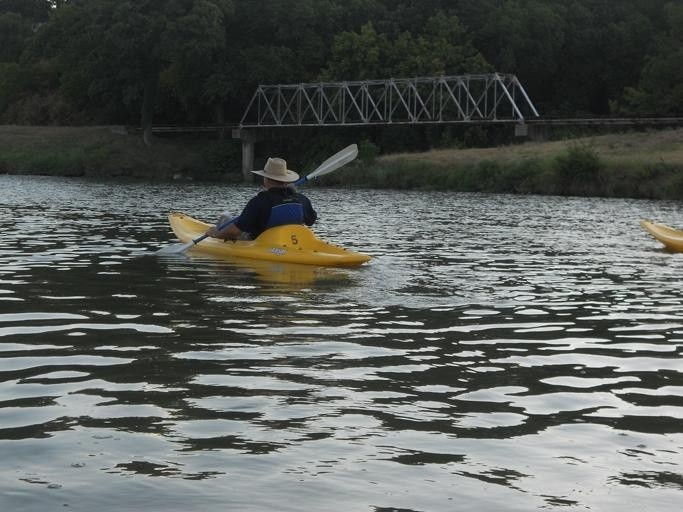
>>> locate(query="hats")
[249,156,301,183]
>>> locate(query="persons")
[206,157,317,241]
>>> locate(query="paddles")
[156,143,358,255]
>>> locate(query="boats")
[179,246,351,289]
[639,219,682,251]
[169,210,371,269]
